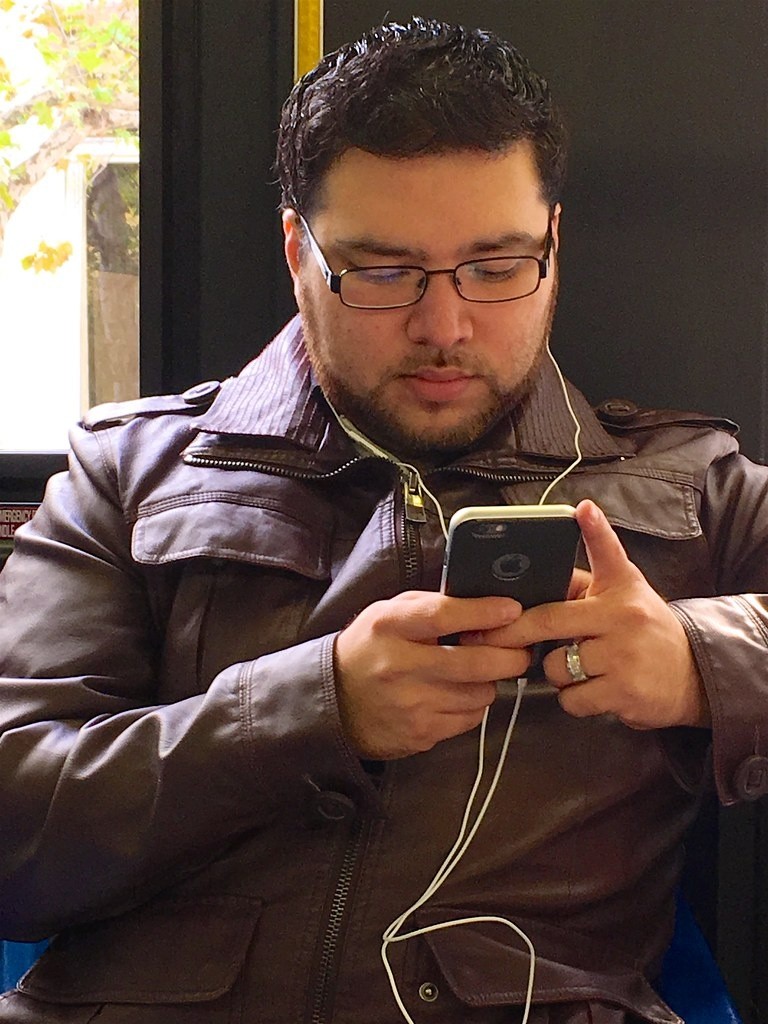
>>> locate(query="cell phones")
[436,502,581,680]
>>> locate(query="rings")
[565,640,589,685]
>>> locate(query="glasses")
[294,200,553,311]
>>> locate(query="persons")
[4,20,767,1024]
